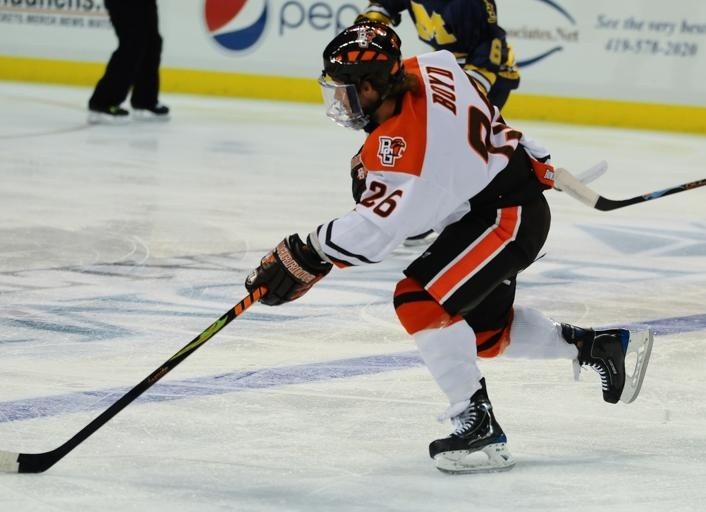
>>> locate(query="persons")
[239,20,634,465]
[83,0,171,118]
[350,0,524,241]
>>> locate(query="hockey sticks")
[547,161,609,194]
[0,282,267,476]
[552,168,706,211]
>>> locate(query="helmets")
[318,21,404,100]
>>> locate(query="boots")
[89,100,169,115]
[429,376,507,459]
[561,322,630,404]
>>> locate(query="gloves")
[244,233,333,306]
[351,144,368,205]
